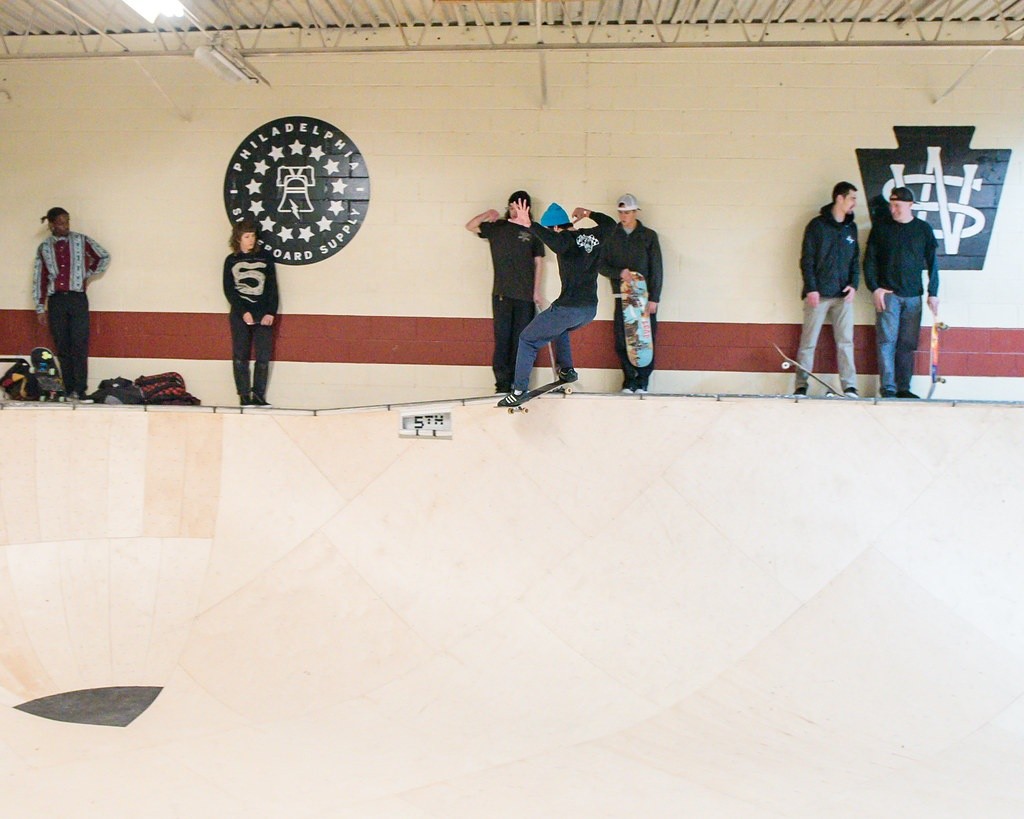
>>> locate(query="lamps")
[193,41,272,88]
[121,0,186,25]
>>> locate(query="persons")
[466,190,545,395]
[498,198,617,406]
[794,181,860,398]
[863,187,938,400]
[33,208,110,404]
[599,193,663,394]
[223,220,279,407]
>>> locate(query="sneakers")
[239,395,256,408]
[251,396,272,408]
[496,390,529,407]
[557,367,578,384]
[843,388,860,401]
[78,393,94,404]
[881,388,899,400]
[897,390,920,401]
[619,384,649,394]
[792,387,807,397]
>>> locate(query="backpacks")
[134,372,184,401]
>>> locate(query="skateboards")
[771,342,842,398]
[29,347,65,403]
[926,315,948,398]
[494,379,572,413]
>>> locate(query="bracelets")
[583,209,587,217]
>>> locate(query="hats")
[540,201,569,228]
[889,186,920,205]
[616,192,643,212]
[508,190,532,209]
